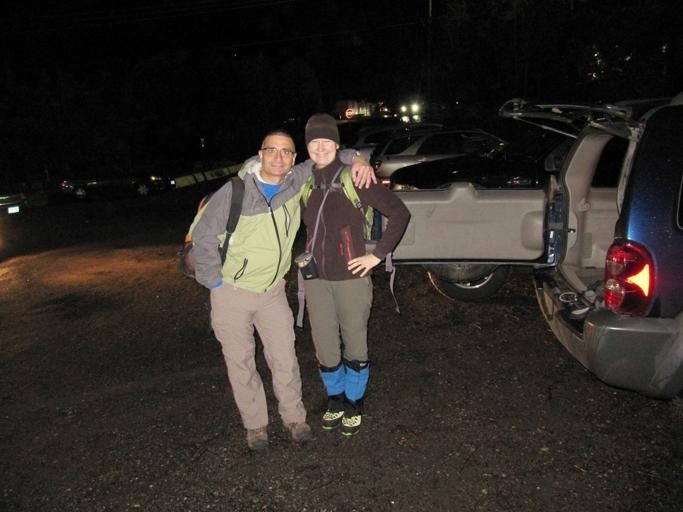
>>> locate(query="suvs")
[374,89,682,400]
[399,95,426,115]
[349,119,443,153]
[370,127,509,187]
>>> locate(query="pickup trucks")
[387,102,580,303]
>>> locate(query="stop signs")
[345,108,352,119]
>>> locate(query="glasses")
[261,146,296,157]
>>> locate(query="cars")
[0,183,27,220]
[61,171,176,200]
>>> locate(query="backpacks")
[178,177,244,279]
[303,164,374,240]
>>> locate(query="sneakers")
[283,420,313,442]
[247,427,269,451]
[322,409,344,429]
[341,416,362,437]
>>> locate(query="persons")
[191,132,377,452]
[238,114,411,436]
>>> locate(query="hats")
[305,114,340,145]
